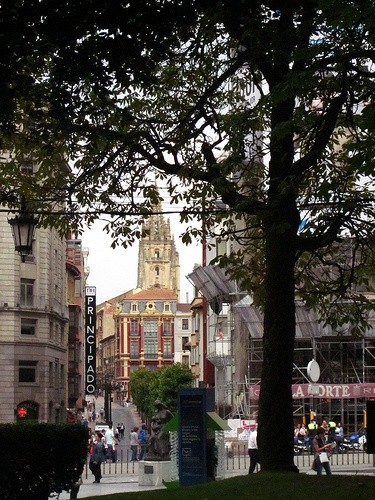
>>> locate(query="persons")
[86,397,147,484]
[312,427,332,476]
[293,418,347,453]
[248,420,261,474]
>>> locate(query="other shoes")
[93,481,100,483]
[96,475,102,479]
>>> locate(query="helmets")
[329,421,336,427]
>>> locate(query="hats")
[97,432,103,438]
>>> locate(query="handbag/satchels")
[319,452,329,463]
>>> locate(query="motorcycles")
[293,429,368,455]
